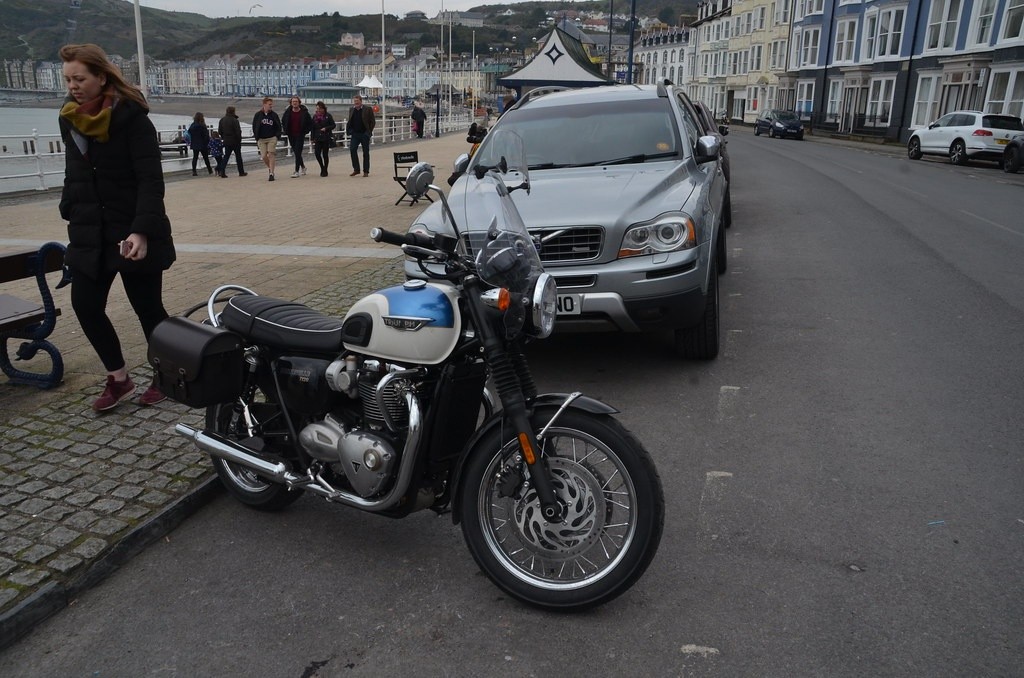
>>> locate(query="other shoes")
[269,173,275,181]
[320,166,328,176]
[192,169,198,176]
[350,170,360,176]
[363,173,368,177]
[220,170,227,178]
[208,167,213,174]
[291,171,299,177]
[301,167,307,175]
[239,172,248,176]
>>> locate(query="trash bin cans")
[331,121,344,146]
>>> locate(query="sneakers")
[139,380,168,405]
[94,374,136,410]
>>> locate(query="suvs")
[405,78,735,363]
[907,110,1024,165]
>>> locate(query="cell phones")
[120,240,133,256]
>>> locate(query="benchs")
[0,242,72,391]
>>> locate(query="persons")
[311,101,336,177]
[345,95,375,177]
[218,106,248,178]
[282,96,312,177]
[503,95,517,114]
[411,105,427,138]
[57,44,177,413]
[252,97,281,181]
[187,112,213,176]
[208,130,224,177]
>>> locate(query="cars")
[754,109,805,139]
[463,97,481,109]
[1002,136,1024,174]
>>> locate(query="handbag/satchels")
[329,135,336,147]
[183,128,191,146]
[411,120,418,131]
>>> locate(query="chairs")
[393,151,434,207]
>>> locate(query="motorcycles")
[718,110,730,125]
[150,131,664,613]
[717,120,731,136]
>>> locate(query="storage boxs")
[147,316,244,408]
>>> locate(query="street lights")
[461,54,479,116]
[489,46,509,78]
[512,36,537,66]
